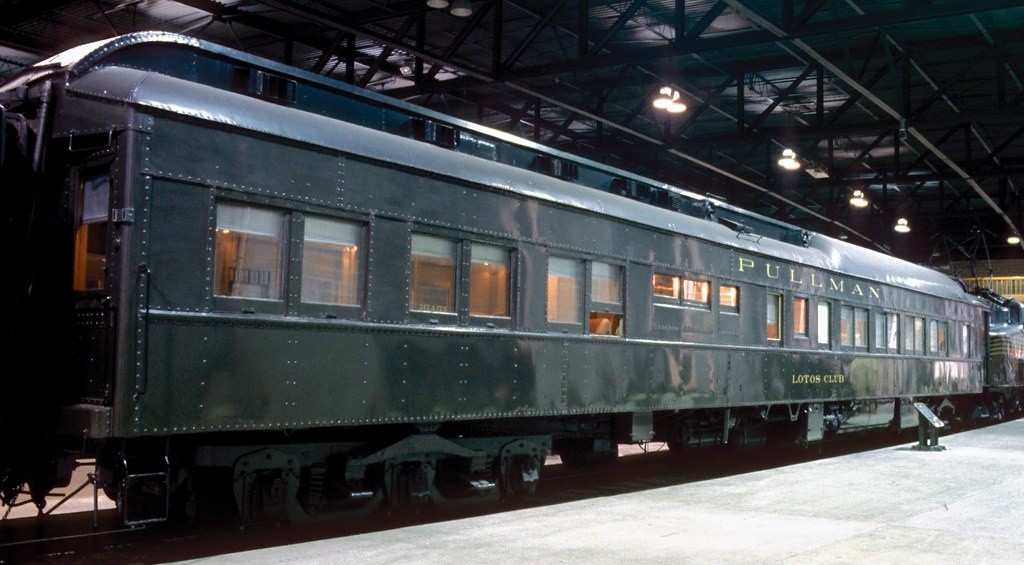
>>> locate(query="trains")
[0,30,1022,549]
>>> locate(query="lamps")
[399,63,412,76]
[426,0,474,17]
[849,189,869,208]
[1007,231,1020,245]
[778,148,801,170]
[653,85,687,114]
[894,217,910,233]
